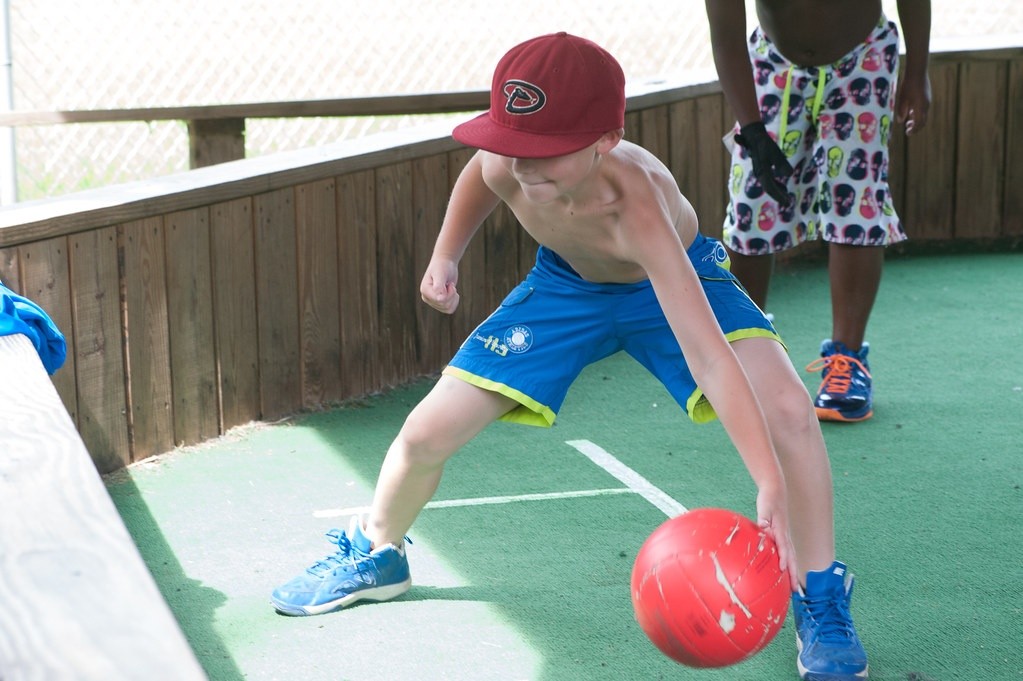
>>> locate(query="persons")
[270,31,869,681]
[704,0,932,423]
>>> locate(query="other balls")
[628,509,792,668]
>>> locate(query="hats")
[451,32,626,158]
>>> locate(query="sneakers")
[805,339,875,422]
[792,560,869,681]
[270,519,413,617]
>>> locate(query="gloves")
[734,122,793,206]
